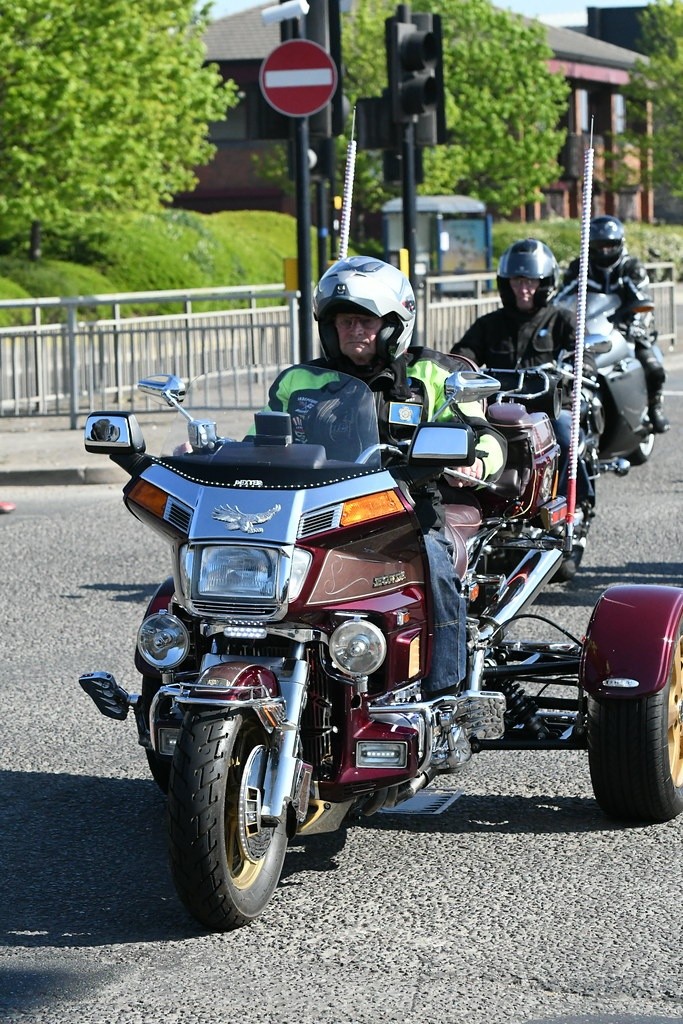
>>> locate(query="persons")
[174,255,508,772]
[447,238,600,535]
[556,215,671,432]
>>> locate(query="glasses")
[508,277,540,287]
[334,316,380,330]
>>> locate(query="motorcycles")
[549,281,656,465]
[80,368,683,931]
[453,333,634,583]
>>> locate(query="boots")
[646,384,670,432]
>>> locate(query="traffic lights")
[385,20,439,123]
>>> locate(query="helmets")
[497,240,558,311]
[590,216,625,268]
[313,256,416,365]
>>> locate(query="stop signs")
[259,39,338,117]
